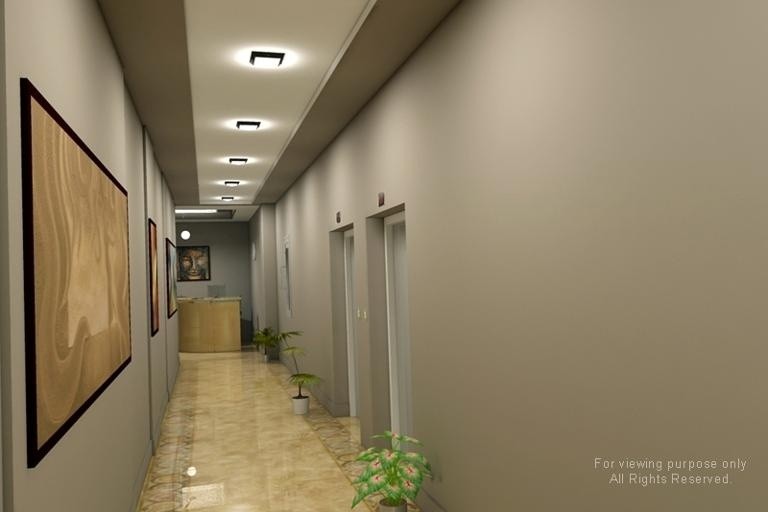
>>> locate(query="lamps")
[219,52,286,201]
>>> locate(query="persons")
[178,247,209,281]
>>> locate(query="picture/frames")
[147,218,178,339]
[17,76,131,471]
[176,245,210,282]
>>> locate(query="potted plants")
[251,327,278,363]
[265,330,325,415]
[349,430,432,512]
